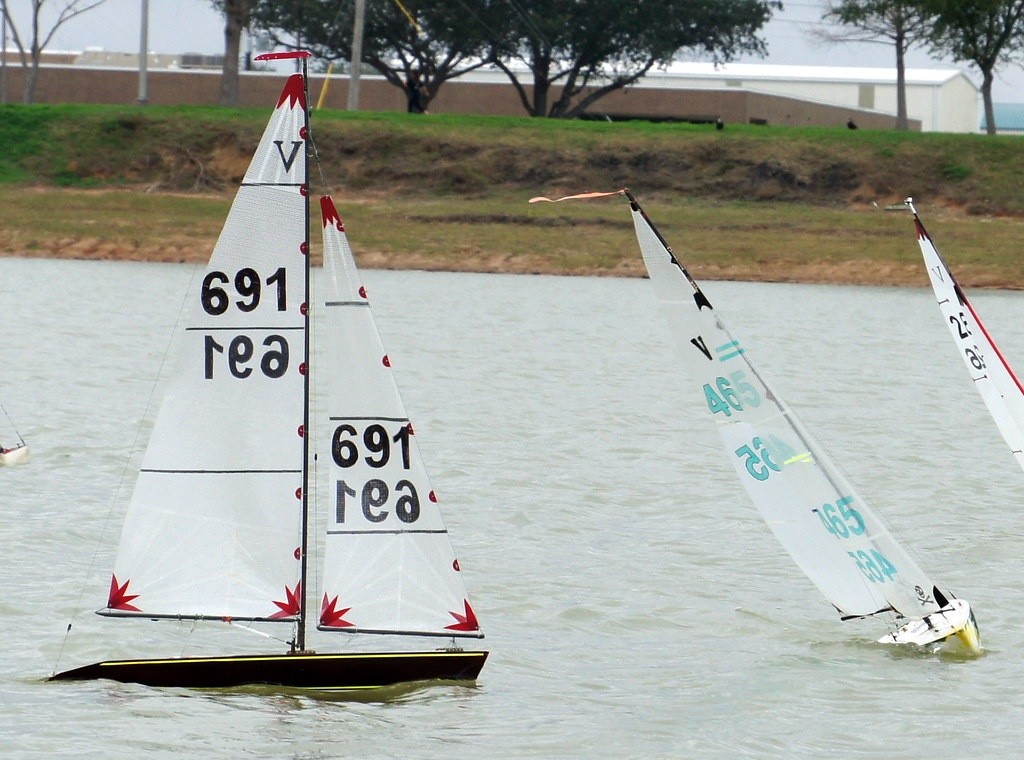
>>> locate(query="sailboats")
[0,407,32,469]
[869,195,1024,474]
[526,184,985,657]
[43,46,496,692]
